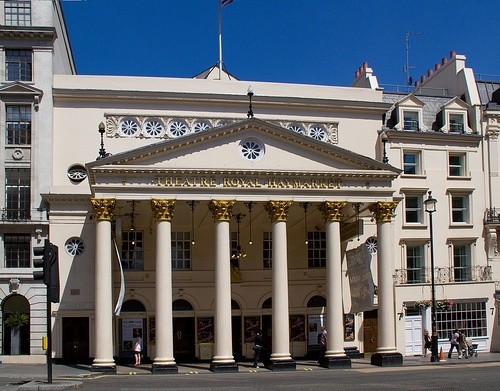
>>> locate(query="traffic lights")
[33,237,52,285]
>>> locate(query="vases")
[419,304,426,310]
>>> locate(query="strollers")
[465,340,479,357]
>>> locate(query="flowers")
[434,298,453,312]
[413,300,431,311]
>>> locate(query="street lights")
[424,190,439,363]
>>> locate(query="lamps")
[243,201,256,244]
[232,212,247,259]
[351,202,363,241]
[299,202,312,244]
[187,200,200,245]
[124,201,142,244]
[247,86,255,119]
[98,122,106,159]
[381,131,389,163]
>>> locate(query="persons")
[133,332,142,366]
[318,327,328,368]
[448,328,463,358]
[424,332,432,352]
[458,330,468,359]
[253,330,263,369]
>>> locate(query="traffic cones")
[439,347,444,360]
